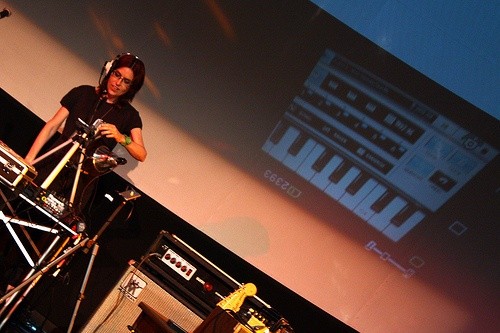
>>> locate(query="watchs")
[121,134,132,147]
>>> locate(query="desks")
[1,191,78,330]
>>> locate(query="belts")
[65,161,90,175]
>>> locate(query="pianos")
[260,47,498,241]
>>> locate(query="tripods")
[28,133,91,208]
[0,199,127,333]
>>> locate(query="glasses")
[112,70,132,86]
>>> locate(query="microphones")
[99,67,108,94]
[0,7,13,19]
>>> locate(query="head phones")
[104,52,140,77]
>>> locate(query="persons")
[0,52,147,303]
